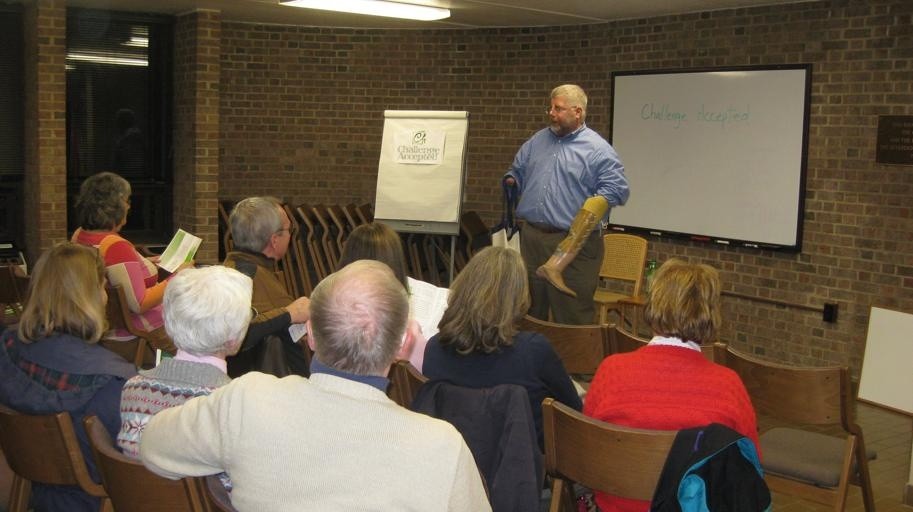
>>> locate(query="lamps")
[64,51,152,67]
[279,0,451,23]
[121,36,147,48]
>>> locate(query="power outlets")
[822,303,838,323]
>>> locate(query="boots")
[536,209,601,299]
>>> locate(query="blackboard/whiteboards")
[604,65,812,254]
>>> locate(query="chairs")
[540,397,678,512]
[198,471,233,511]
[714,341,875,512]
[342,203,363,229]
[83,412,209,512]
[218,202,234,256]
[313,207,341,274]
[0,264,23,324]
[327,204,345,264]
[1,403,110,512]
[102,284,162,369]
[543,232,650,329]
[607,323,718,366]
[12,264,35,302]
[354,203,374,224]
[455,236,465,270]
[391,358,427,410]
[284,203,313,299]
[433,235,459,282]
[296,206,328,283]
[282,250,300,301]
[270,263,280,272]
[406,233,424,281]
[460,210,489,261]
[422,234,440,288]
[517,314,607,380]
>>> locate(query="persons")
[223,196,311,381]
[117,265,253,501]
[1,242,139,512]
[422,246,584,490]
[502,84,629,383]
[583,258,763,511]
[338,223,428,376]
[140,260,492,511]
[70,173,196,365]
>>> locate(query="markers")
[613,227,624,230]
[715,240,729,244]
[692,237,709,240]
[650,232,661,235]
[744,244,758,248]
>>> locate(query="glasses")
[544,105,562,115]
[277,227,293,234]
[251,307,257,320]
[123,199,131,205]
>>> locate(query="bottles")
[645,259,656,295]
